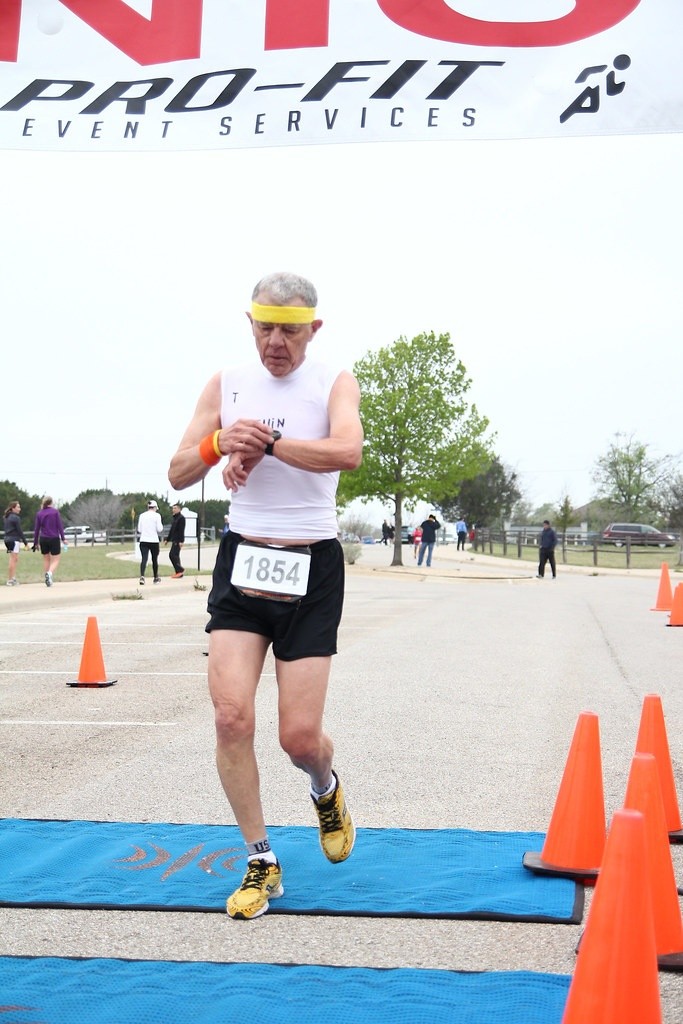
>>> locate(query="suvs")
[602,522,676,549]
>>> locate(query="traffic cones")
[633,695,682,846]
[65,616,119,688]
[667,582,683,617]
[559,808,667,1024]
[665,587,683,627]
[649,562,673,612]
[521,710,608,880]
[573,752,683,974]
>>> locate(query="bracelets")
[200,430,224,467]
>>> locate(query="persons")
[169,273,365,919]
[418,515,441,567]
[2,501,30,586]
[413,525,422,558]
[456,518,467,551]
[380,520,395,545]
[138,500,163,585]
[34,495,68,587]
[407,523,414,545]
[223,515,229,533]
[536,520,558,578]
[164,504,186,578]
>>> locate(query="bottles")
[63,543,67,552]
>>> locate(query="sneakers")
[227,856,285,921]
[309,768,355,864]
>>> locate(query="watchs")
[265,430,282,456]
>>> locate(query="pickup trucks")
[59,526,107,543]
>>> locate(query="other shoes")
[154,578,161,583]
[172,570,186,578]
[140,576,145,584]
[536,575,543,578]
[45,572,52,587]
[7,578,16,586]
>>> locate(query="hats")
[148,500,159,510]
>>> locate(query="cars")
[336,524,458,546]
